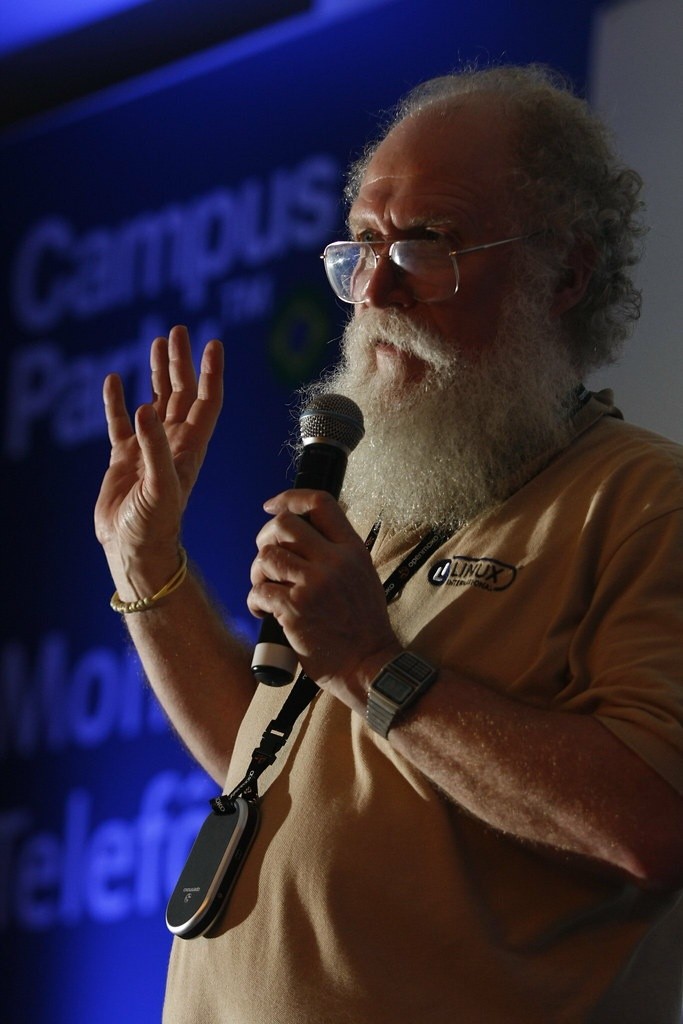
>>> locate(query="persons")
[95,64,683,1024]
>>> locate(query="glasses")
[319,227,553,305]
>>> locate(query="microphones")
[252,394,365,689]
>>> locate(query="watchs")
[364,650,436,740]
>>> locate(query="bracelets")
[110,541,192,613]
